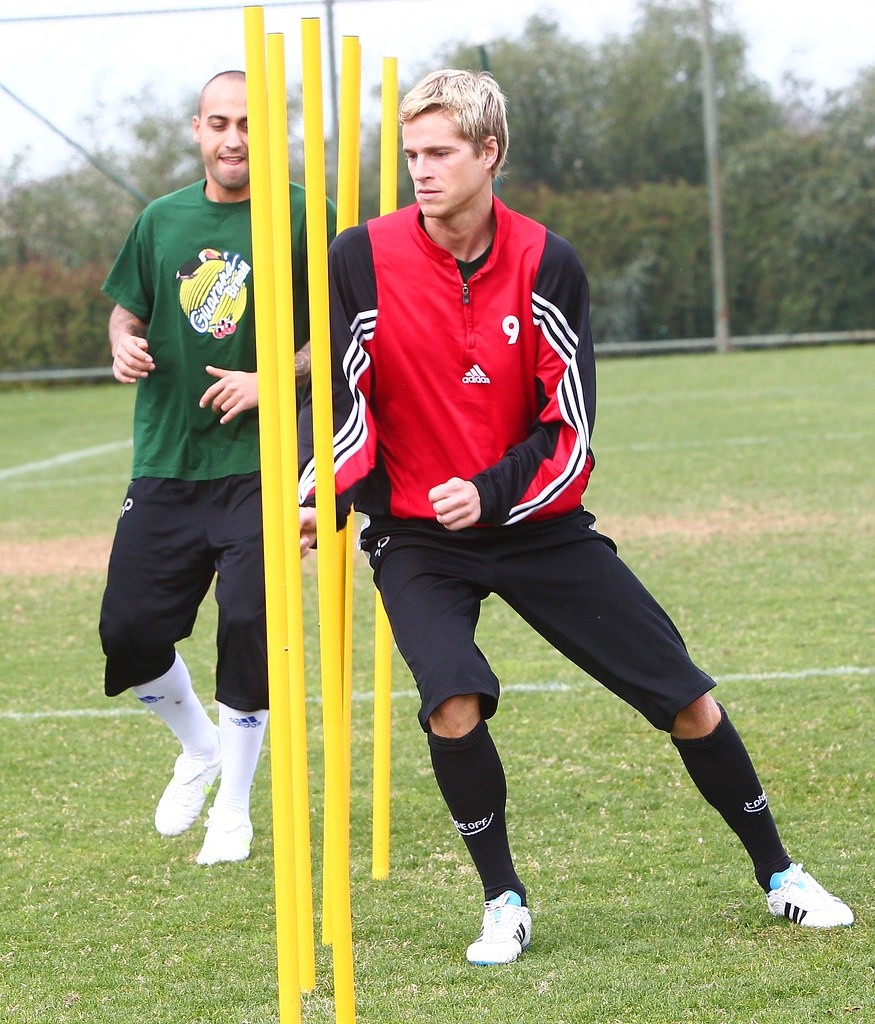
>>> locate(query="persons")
[100,72,336,863]
[296,69,853,965]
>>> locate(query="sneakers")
[154,740,224,838]
[466,890,532,966]
[197,802,255,866]
[765,861,855,931]
